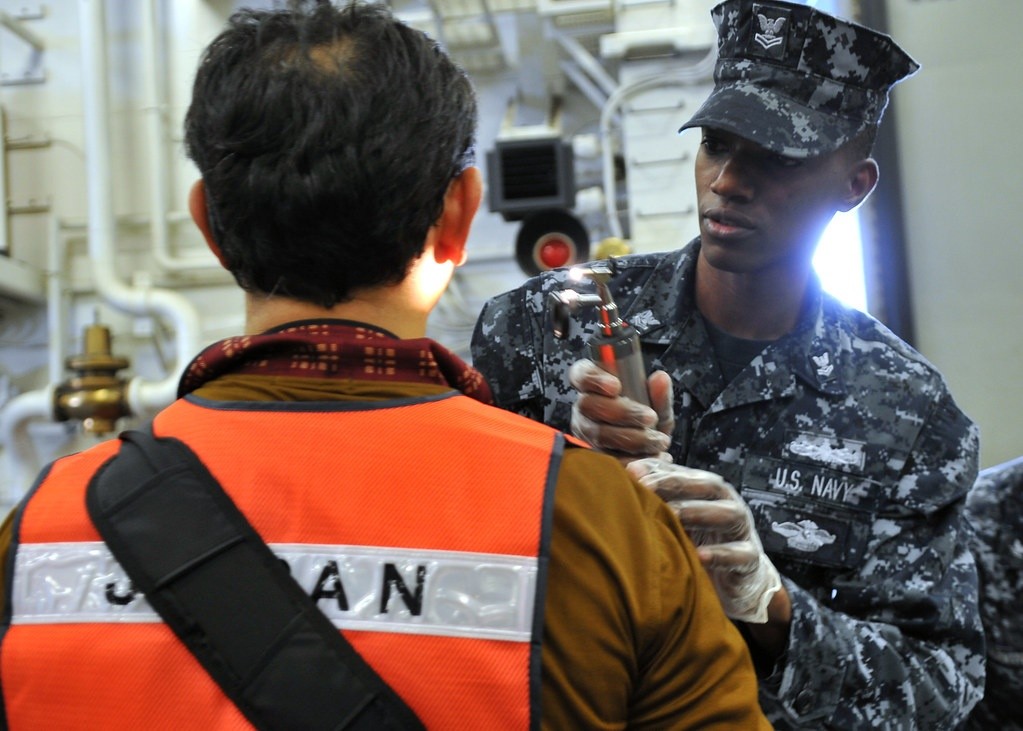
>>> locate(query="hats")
[677,0,920,159]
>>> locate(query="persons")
[0,2,776,731]
[470,0,1023,731]
